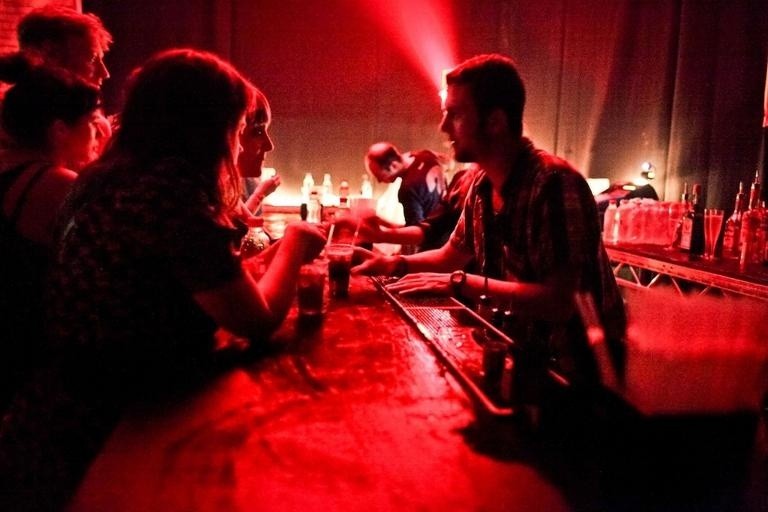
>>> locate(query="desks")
[71,272,658,511]
[604,241,768,300]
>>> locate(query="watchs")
[447,269,467,298]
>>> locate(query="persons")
[15,11,118,164]
[324,54,630,388]
[2,41,327,511]
[1,50,106,404]
[324,166,482,258]
[224,80,279,250]
[363,143,449,230]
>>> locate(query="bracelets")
[483,273,489,296]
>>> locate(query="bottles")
[301,170,373,199]
[721,165,768,266]
[476,293,521,334]
[680,182,705,254]
[602,197,691,244]
[240,216,270,258]
[334,198,353,218]
[306,190,322,224]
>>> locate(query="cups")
[699,207,724,260]
[325,242,354,297]
[508,343,553,389]
[296,270,322,314]
[483,341,505,381]
[260,168,277,183]
